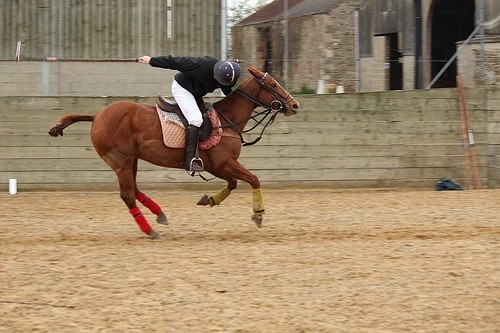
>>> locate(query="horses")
[47,66,300,240]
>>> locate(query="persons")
[138,54,242,173]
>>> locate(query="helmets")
[214,59,241,85]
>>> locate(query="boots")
[185,124,203,171]
